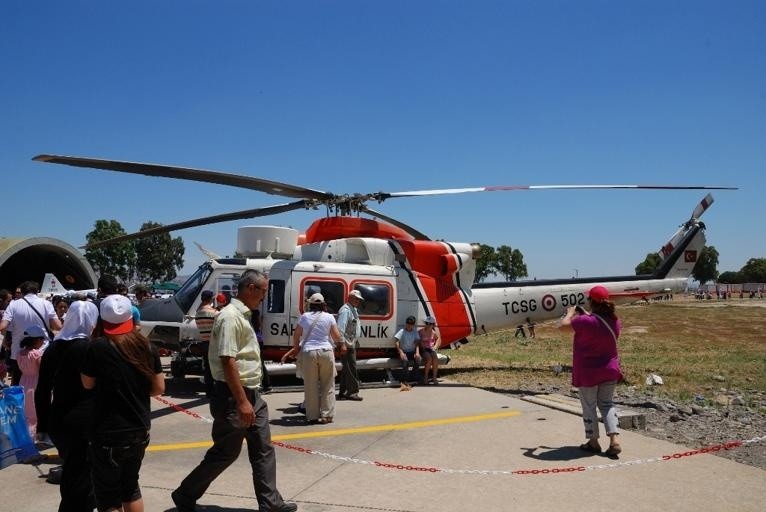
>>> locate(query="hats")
[349,289,365,300]
[100,294,135,335]
[22,324,51,341]
[424,317,436,324]
[306,293,325,304]
[583,286,610,303]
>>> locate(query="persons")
[194,290,220,398]
[0,273,166,512]
[527,324,536,339]
[417,316,440,386]
[251,309,273,394]
[292,292,348,424]
[651,287,764,300]
[558,285,624,457]
[393,314,421,385]
[170,268,297,512]
[215,292,229,311]
[514,324,526,338]
[336,289,364,401]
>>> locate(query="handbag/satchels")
[47,329,55,341]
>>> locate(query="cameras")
[575,305,581,311]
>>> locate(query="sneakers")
[171,486,196,512]
[400,378,423,385]
[432,377,438,385]
[424,376,429,384]
[309,419,318,423]
[321,418,332,423]
[259,503,298,512]
[338,393,346,399]
[580,441,602,453]
[606,444,622,456]
[346,393,363,400]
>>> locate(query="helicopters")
[33,267,180,301]
[29,150,739,389]
